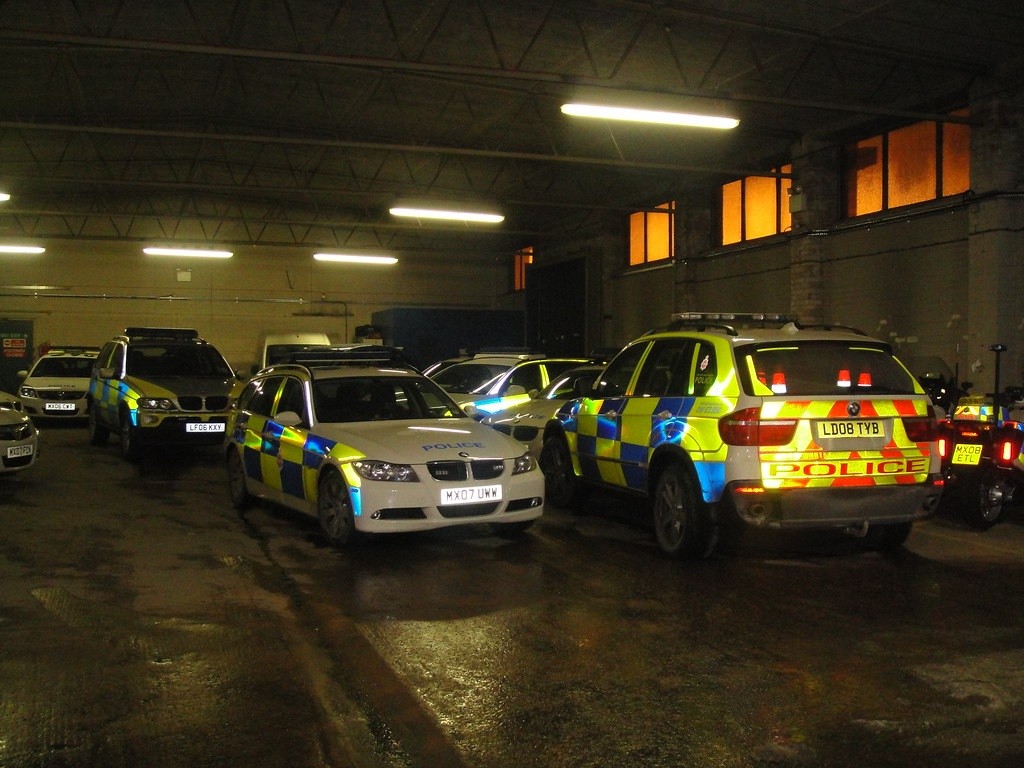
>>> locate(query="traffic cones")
[753,355,873,393]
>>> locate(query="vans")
[250,331,332,386]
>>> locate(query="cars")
[478,362,672,482]
[390,357,472,405]
[0,391,31,419]
[0,407,39,475]
[17,350,101,421]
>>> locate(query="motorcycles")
[950,345,1024,531]
[905,351,974,513]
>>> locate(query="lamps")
[142,242,235,260]
[0,238,47,255]
[387,198,505,224]
[557,84,741,131]
[312,247,400,265]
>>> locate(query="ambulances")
[86,325,246,460]
[219,350,546,551]
[541,314,945,573]
[394,353,608,427]
